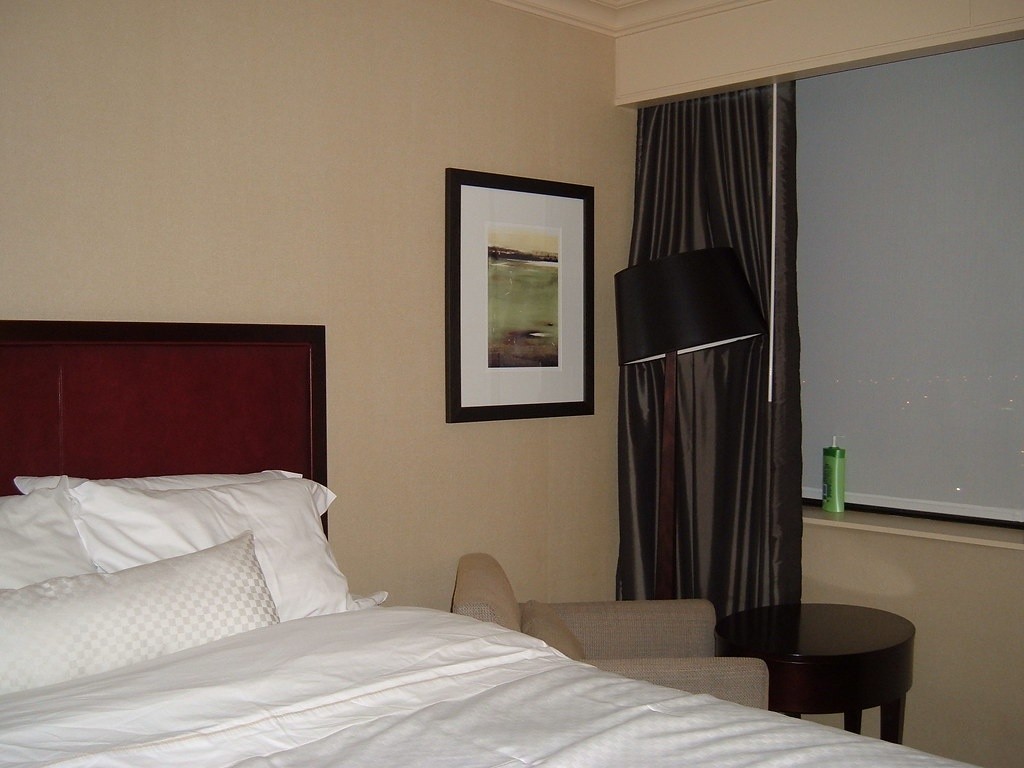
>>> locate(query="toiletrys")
[821,434,848,514]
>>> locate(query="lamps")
[614,244,767,599]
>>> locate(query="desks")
[714,604,916,746]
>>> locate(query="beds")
[0,320,984,768]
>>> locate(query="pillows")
[63,477,357,622]
[520,600,586,661]
[13,469,302,494]
[0,475,99,589]
[0,530,281,695]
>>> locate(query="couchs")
[451,551,770,712]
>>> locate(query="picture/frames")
[444,167,595,424]
